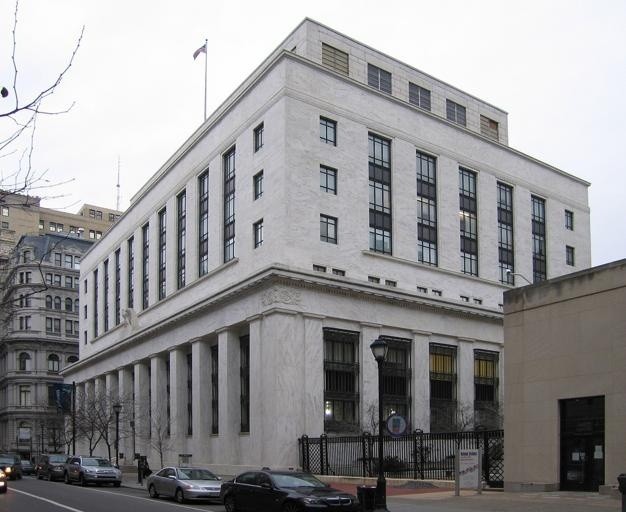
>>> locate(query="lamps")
[504,268,533,285]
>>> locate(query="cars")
[147,467,224,504]
[220,470,357,512]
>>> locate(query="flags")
[193,45,205,61]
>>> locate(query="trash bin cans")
[357,485,378,512]
[617,474,626,512]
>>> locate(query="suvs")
[0,452,122,494]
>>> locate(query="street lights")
[113,402,122,469]
[40,420,45,454]
